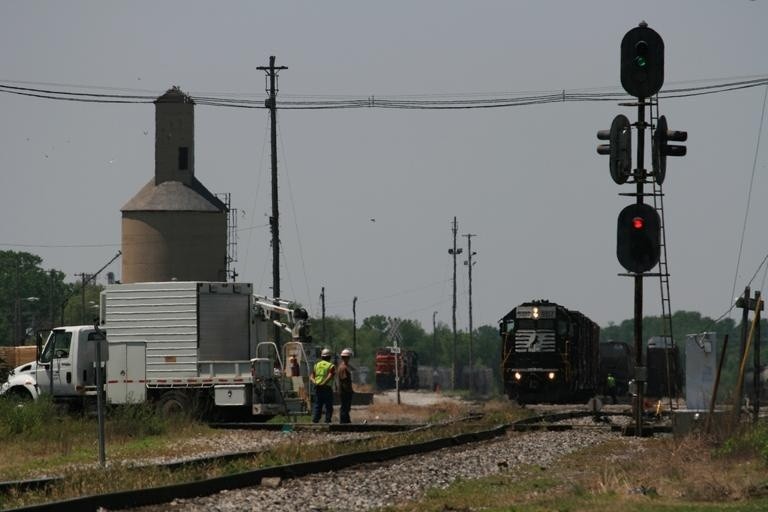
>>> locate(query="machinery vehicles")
[372,346,420,390]
[254,290,375,415]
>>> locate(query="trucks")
[1,275,312,429]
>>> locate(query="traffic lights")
[594,114,634,185]
[619,20,665,98]
[618,203,662,273]
[653,115,689,185]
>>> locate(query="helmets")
[320,348,333,357]
[340,348,352,358]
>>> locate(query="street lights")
[80,299,100,325]
[5,295,41,346]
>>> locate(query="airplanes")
[498,299,643,406]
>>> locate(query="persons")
[338,349,355,423]
[289,354,298,375]
[308,349,335,423]
[607,373,618,405]
